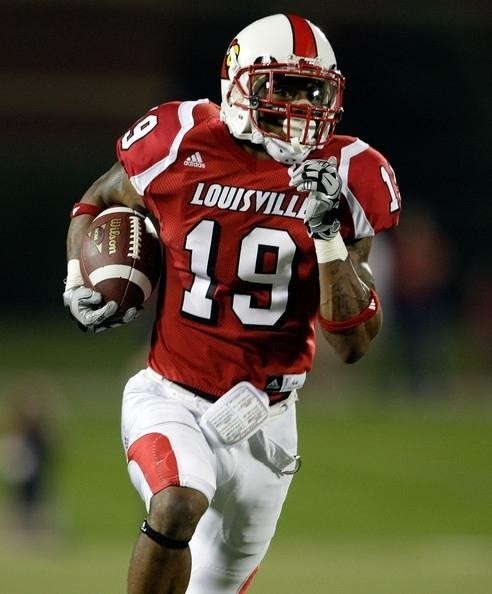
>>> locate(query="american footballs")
[79,202,162,322]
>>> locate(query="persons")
[63,14,402,593]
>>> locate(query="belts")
[171,380,292,408]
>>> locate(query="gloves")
[287,157,342,240]
[63,286,141,335]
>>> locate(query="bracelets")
[313,231,348,265]
[67,259,85,289]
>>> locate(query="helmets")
[219,11,347,165]
